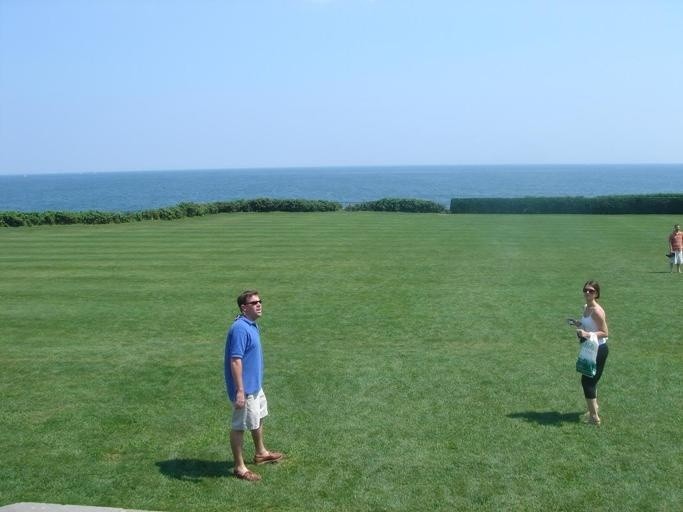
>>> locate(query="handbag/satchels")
[575,332,598,378]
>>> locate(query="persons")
[666,223,682,274]
[221,290,284,483]
[567,280,610,425]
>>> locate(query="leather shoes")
[254,452,283,465]
[235,470,261,480]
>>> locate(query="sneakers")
[582,416,599,424]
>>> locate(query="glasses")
[582,289,595,294]
[247,300,262,305]
